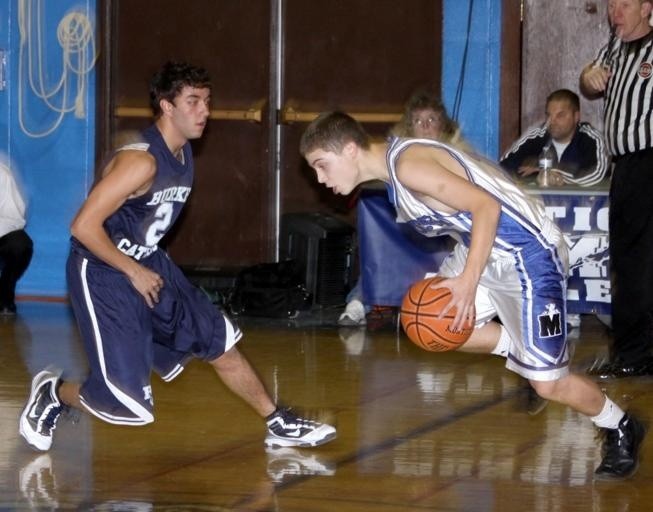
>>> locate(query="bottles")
[537,146,554,190]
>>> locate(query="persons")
[18,64,338,452]
[495,89,610,188]
[338,94,473,328]
[299,112,645,481]
[0,162,33,313]
[580,1,653,379]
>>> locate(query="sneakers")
[263,407,337,447]
[338,301,368,326]
[593,412,648,482]
[18,372,68,452]
[527,341,576,415]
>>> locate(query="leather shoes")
[589,362,647,380]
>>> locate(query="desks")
[522,177,611,316]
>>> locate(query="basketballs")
[400,277,476,352]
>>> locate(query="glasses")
[410,116,439,128]
